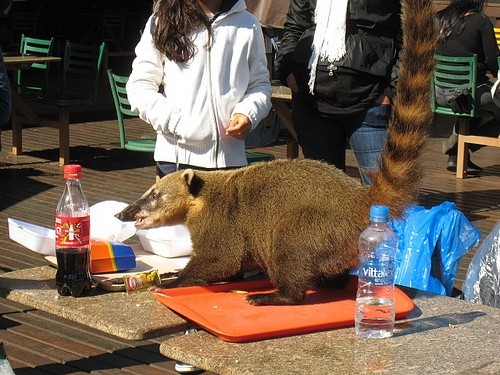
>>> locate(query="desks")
[159,284,500,375]
[3,52,63,64]
[0,266,194,341]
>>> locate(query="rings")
[238,132,241,135]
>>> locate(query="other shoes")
[447,151,483,177]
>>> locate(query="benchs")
[434,53,500,179]
[11,33,300,165]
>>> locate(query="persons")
[126,0,273,180]
[276,0,404,184]
[433,0,500,174]
[0,47,11,122]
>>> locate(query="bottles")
[353,206,397,339]
[53,164,93,297]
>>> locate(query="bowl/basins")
[7,219,58,256]
[136,224,193,258]
